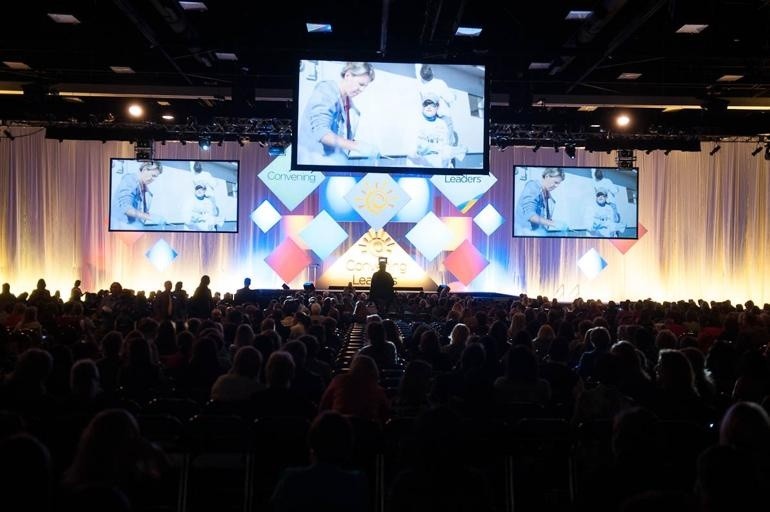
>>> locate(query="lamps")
[161,130,288,156]
[3,126,14,141]
[497,135,765,159]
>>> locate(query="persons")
[516,167,565,236]
[110,160,165,229]
[1,275,769,512]
[297,61,369,167]
[180,183,217,231]
[193,162,221,217]
[418,65,460,149]
[588,185,615,238]
[593,169,622,223]
[371,262,395,313]
[408,95,451,169]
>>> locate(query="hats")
[194,184,207,191]
[595,188,608,197]
[422,93,440,107]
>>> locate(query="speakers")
[304,281,316,291]
[437,284,450,294]
